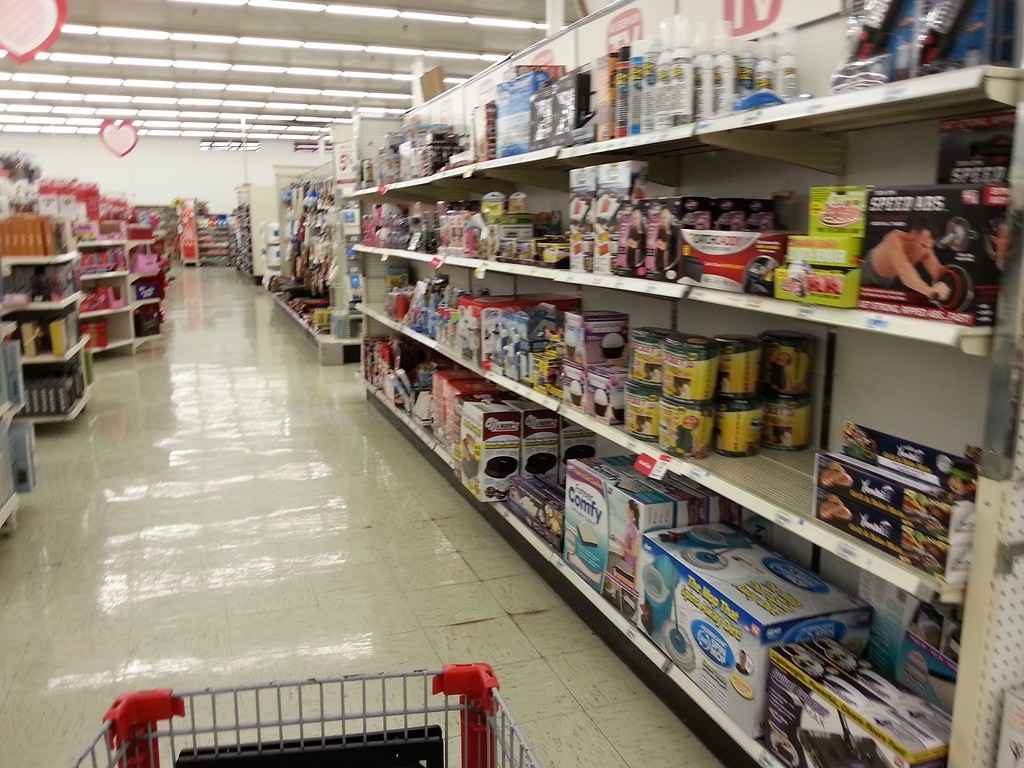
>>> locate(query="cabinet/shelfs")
[356,2,1023,767]
[1,260,38,538]
[1,168,96,424]
[75,151,361,366]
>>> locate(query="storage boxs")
[1,193,95,415]
[0,339,37,495]
[356,65,1011,766]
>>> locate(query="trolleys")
[65,663,544,768]
